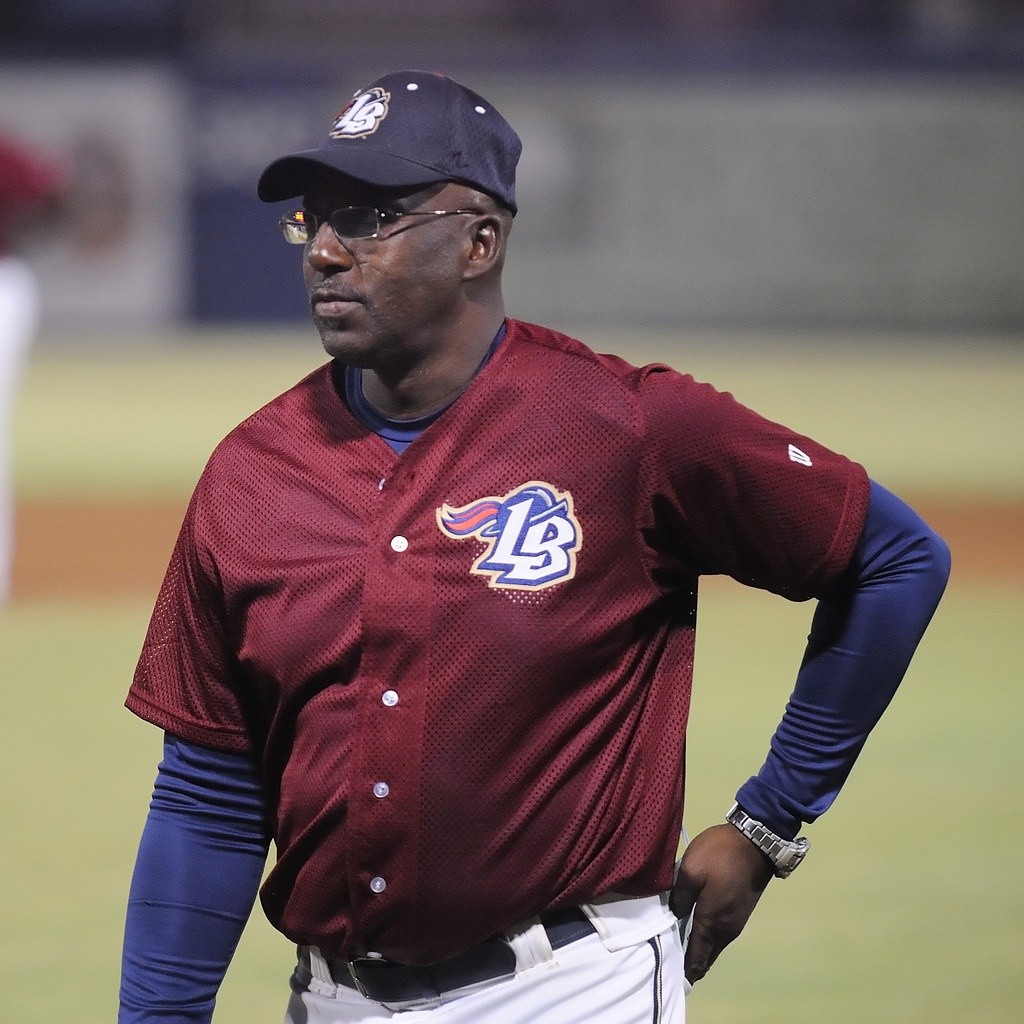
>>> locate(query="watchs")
[723,800,809,879]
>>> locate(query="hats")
[257,71,523,219]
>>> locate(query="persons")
[120,72,955,1024]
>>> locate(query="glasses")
[278,206,483,245]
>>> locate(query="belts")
[326,904,598,1004]
